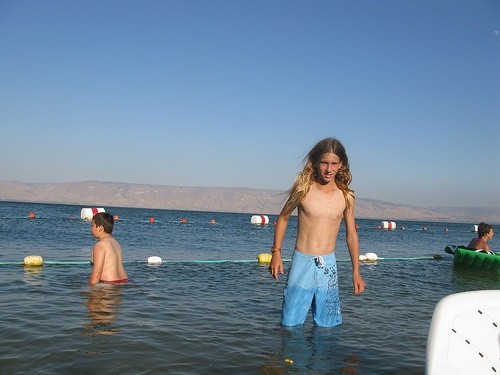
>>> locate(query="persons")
[270,138,366,327]
[468,222,494,250]
[88,212,129,284]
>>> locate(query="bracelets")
[271,247,282,253]
